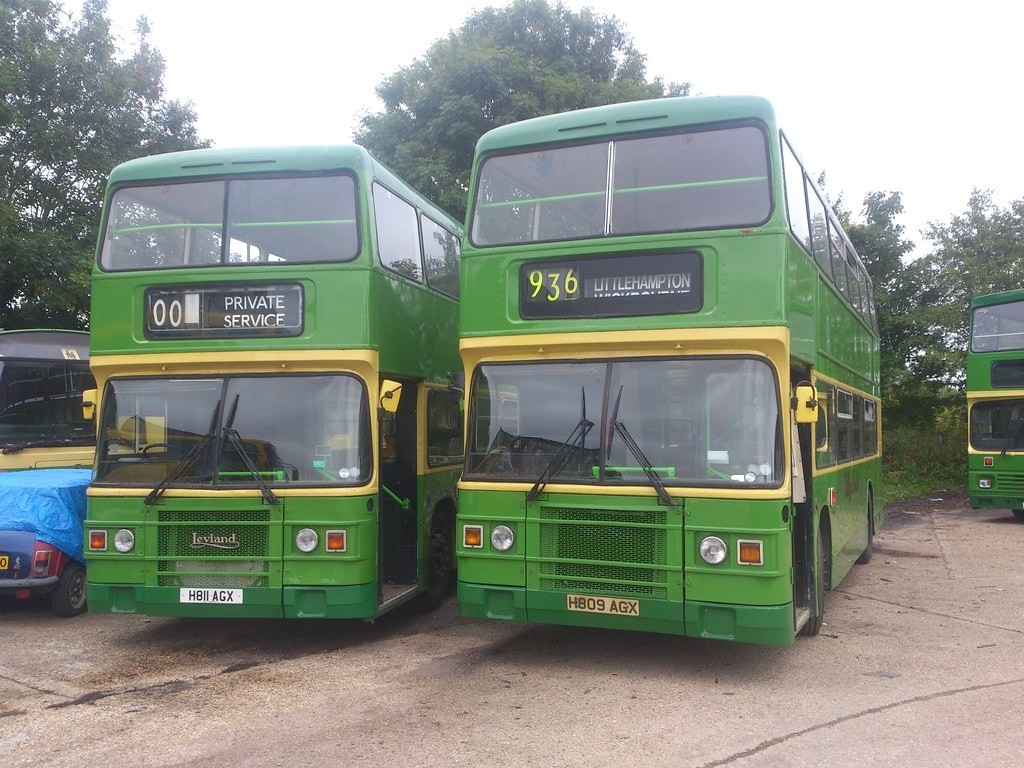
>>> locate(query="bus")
[0,327,167,617]
[71,141,523,626]
[963,289,1023,525]
[451,91,884,647]
